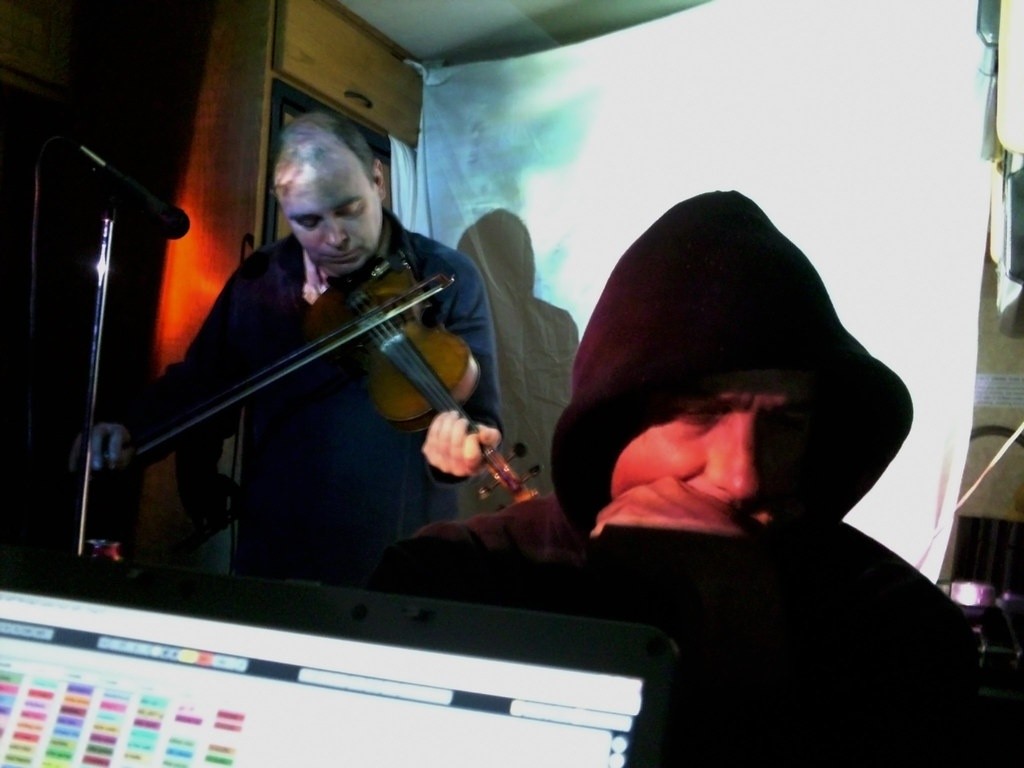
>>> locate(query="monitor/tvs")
[0,543,681,768]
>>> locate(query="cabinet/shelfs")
[233,3,424,148]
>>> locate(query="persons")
[72,108,502,592]
[347,190,1024,768]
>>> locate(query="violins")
[298,250,547,507]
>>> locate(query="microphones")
[72,145,189,239]
